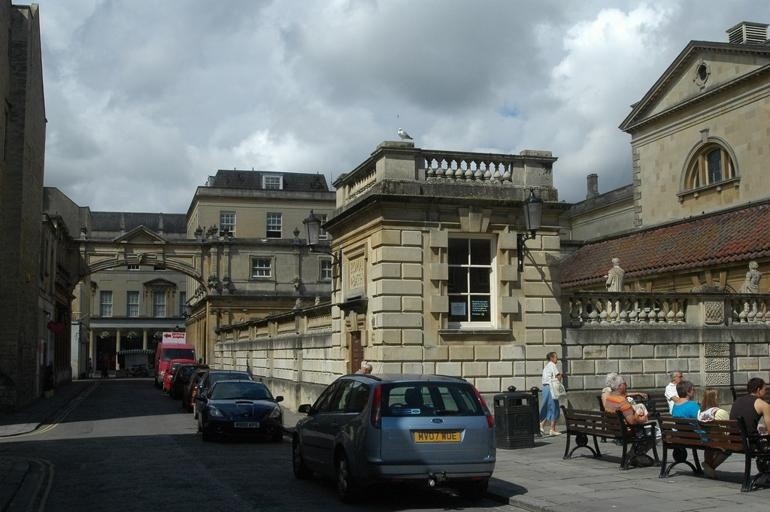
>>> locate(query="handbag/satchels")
[550,378,566,400]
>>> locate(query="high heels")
[540,423,544,432]
[550,430,561,435]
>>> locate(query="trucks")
[154,342,196,388]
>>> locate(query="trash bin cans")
[494,392,536,450]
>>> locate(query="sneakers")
[702,462,716,478]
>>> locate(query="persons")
[605,257,625,292]
[729,378,770,488]
[697,387,732,478]
[663,369,711,444]
[539,350,564,437]
[743,259,761,294]
[600,370,650,467]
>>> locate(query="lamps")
[514,184,547,273]
[300,207,343,282]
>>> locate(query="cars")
[162,358,284,442]
[292,373,498,506]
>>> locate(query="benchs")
[555,381,770,494]
